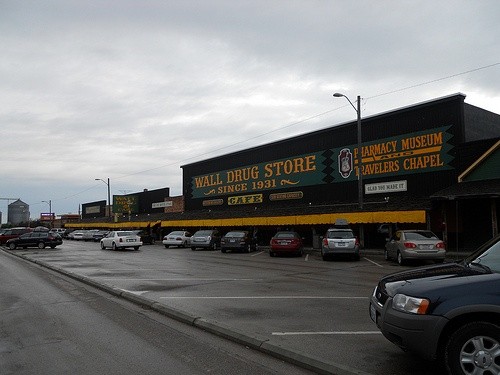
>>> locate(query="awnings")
[63,197,429,228]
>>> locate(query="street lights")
[332,93,365,211]
[95,177,111,217]
[41,200,53,222]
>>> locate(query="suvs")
[321,228,359,261]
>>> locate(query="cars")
[66,230,143,250]
[162,230,192,248]
[369,236,500,375]
[385,229,445,265]
[222,231,256,253]
[191,229,221,250]
[0,226,65,249]
[270,231,303,257]
